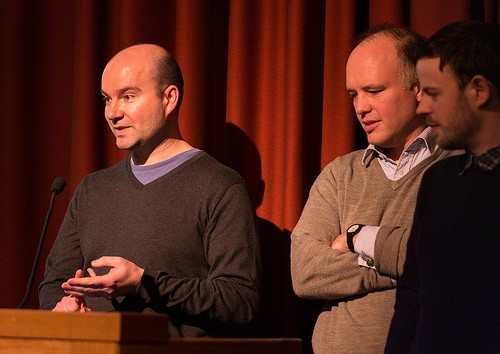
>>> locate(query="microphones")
[18,176,65,309]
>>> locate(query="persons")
[38,43,270,337]
[290,23,465,353]
[384,20,500,354]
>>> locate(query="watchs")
[346,223,366,253]
